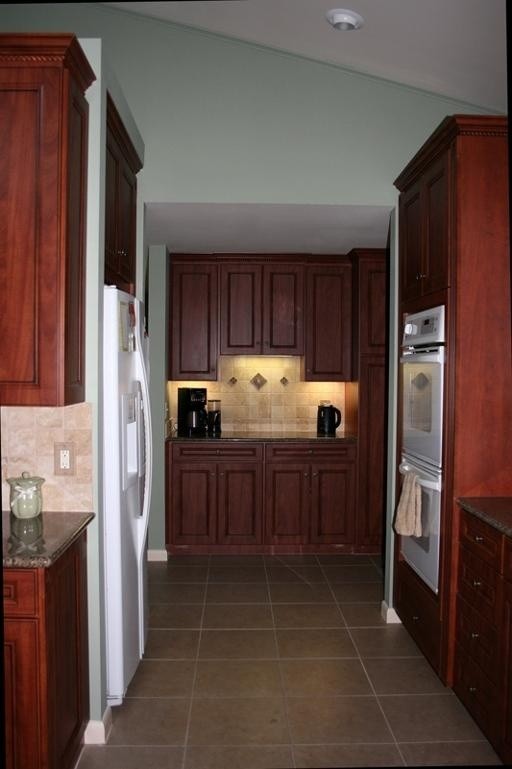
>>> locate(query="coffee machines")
[177,388,207,438]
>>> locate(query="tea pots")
[318,400,342,437]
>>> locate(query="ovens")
[399,453,447,597]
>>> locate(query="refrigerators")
[104,285,153,706]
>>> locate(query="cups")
[208,400,222,437]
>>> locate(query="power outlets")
[52,442,77,477]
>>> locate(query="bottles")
[8,522,45,556]
[7,472,45,519]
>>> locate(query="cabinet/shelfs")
[344,246,385,555]
[164,440,357,555]
[221,253,305,357]
[105,90,144,297]
[0,29,99,408]
[392,110,510,303]
[168,253,221,382]
[4,528,92,769]
[453,509,507,764]
[301,252,355,383]
[393,559,447,680]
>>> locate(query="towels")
[393,473,423,540]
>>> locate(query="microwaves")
[399,305,444,468]
